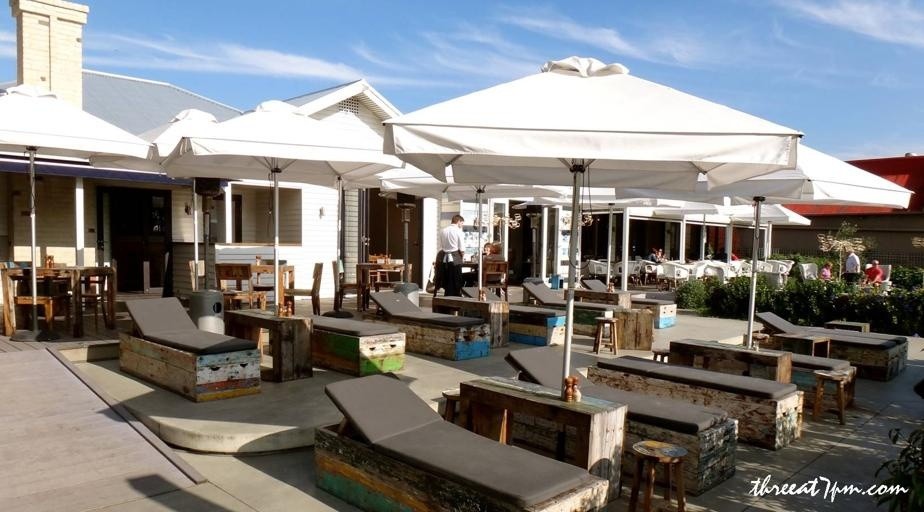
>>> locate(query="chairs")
[84,283,909,512]
[1,258,896,337]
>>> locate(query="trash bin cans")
[190,289,225,335]
